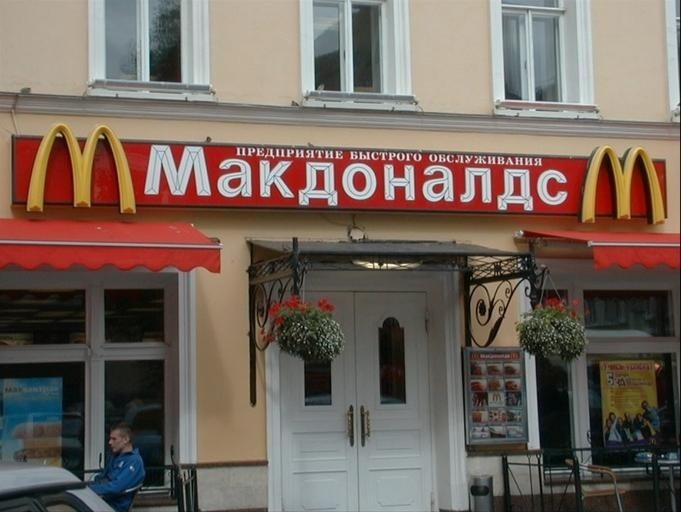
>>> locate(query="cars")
[0,464,116,512]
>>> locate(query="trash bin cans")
[471,474,493,511]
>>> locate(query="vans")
[1,411,83,463]
[121,401,164,472]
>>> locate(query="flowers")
[266,295,347,366]
[516,297,591,361]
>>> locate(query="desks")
[636,454,681,510]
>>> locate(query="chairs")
[109,483,145,512]
[563,454,623,511]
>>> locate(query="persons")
[604,399,661,448]
[84,420,148,512]
